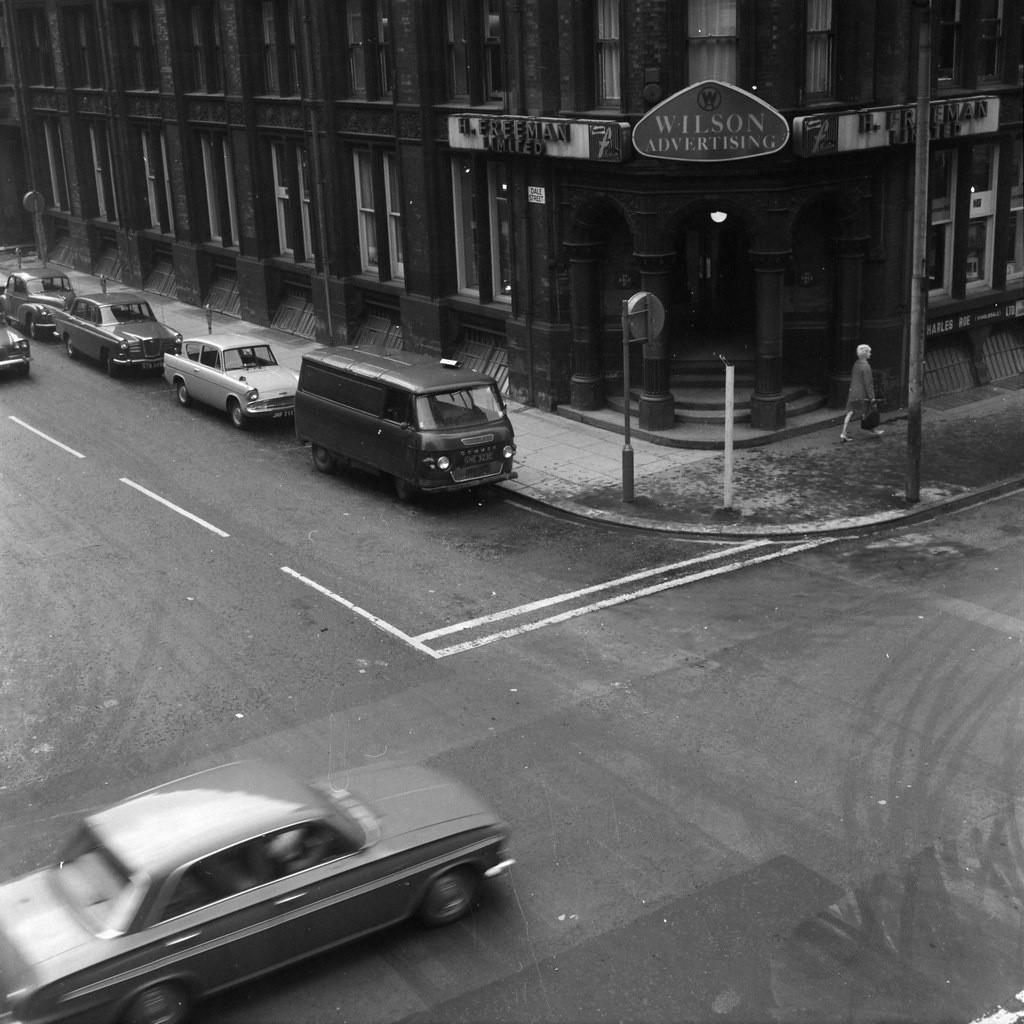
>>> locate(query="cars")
[0,301,30,380]
[0,268,77,339]
[0,757,507,1024]
[54,292,184,379]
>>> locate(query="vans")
[295,344,518,502]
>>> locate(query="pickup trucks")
[163,335,301,429]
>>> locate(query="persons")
[839,343,885,442]
[263,820,333,880]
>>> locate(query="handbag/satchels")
[861,397,880,430]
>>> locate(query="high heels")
[839,433,853,443]
[869,427,884,437]
